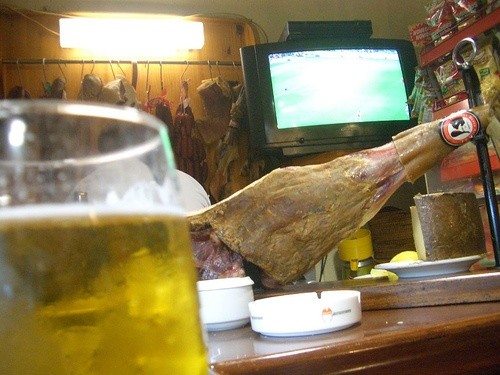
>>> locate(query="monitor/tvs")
[239,38,419,157]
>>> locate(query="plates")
[374,256,486,277]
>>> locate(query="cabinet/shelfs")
[418,0,500,257]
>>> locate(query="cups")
[0,99,211,375]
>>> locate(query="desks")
[202,291,500,375]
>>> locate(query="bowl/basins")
[195,277,253,333]
